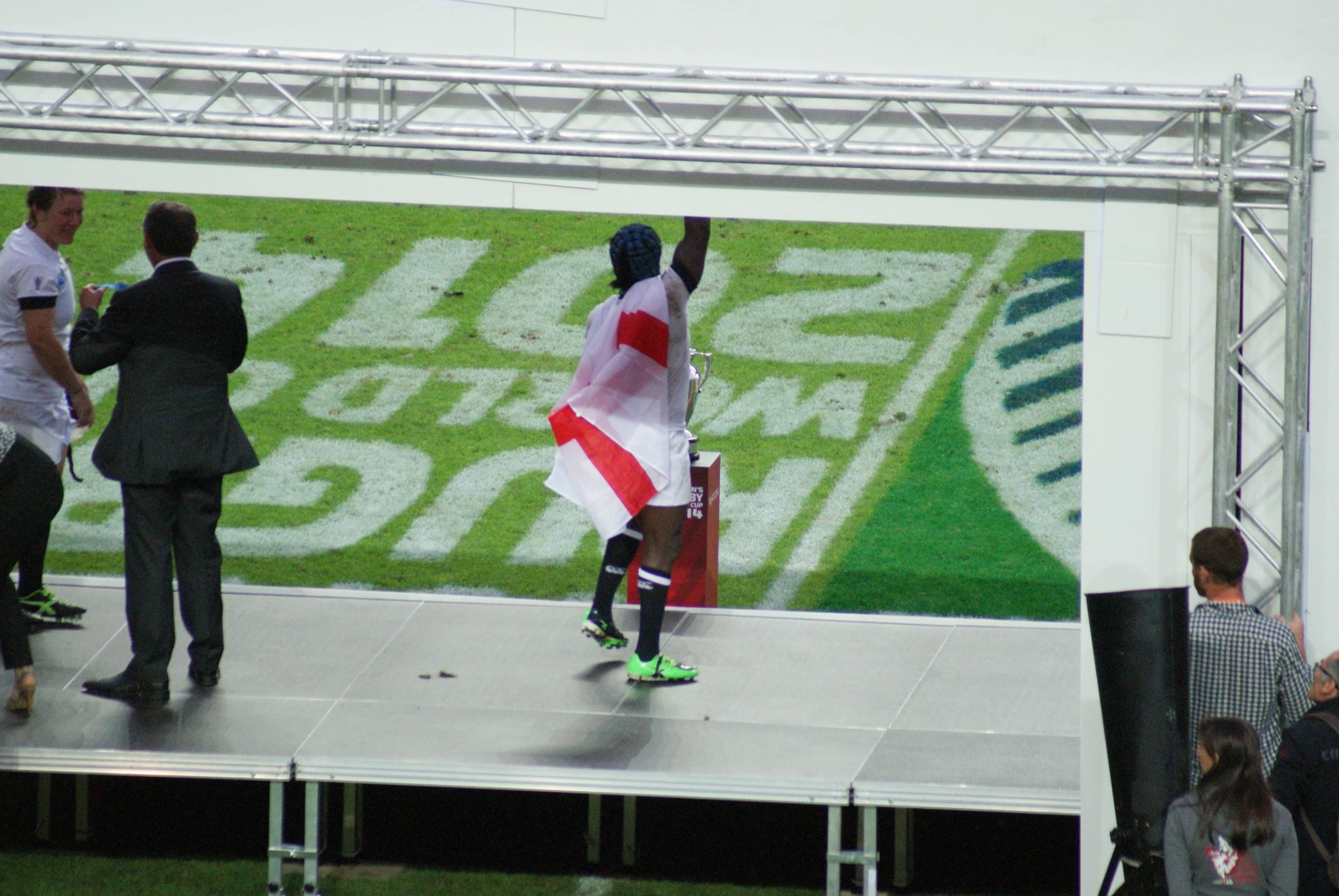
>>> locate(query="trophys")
[687,346,710,459]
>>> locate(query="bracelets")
[70,379,84,393]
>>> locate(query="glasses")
[1312,662,1339,691]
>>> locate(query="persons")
[1163,719,1299,896]
[543,217,709,686]
[0,424,65,715]
[1188,527,1312,797]
[71,201,260,705]
[1269,653,1339,896]
[0,185,89,622]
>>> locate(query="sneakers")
[581,607,629,648]
[627,652,699,681]
[16,584,86,623]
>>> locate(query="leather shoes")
[197,666,220,688]
[82,671,168,702]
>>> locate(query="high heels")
[6,671,37,712]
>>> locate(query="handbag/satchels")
[1328,846,1339,889]
